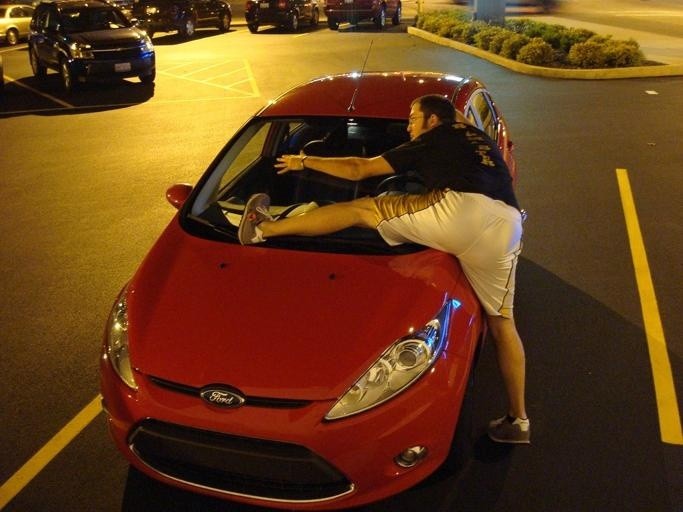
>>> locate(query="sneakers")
[237,192,275,247]
[486,412,532,445]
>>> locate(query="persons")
[237,92,531,446]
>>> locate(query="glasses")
[406,116,431,122]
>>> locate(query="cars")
[98,70,517,511]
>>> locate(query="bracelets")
[299,155,307,170]
